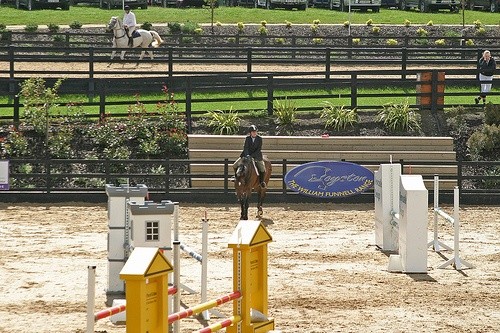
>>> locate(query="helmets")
[124,6,130,10]
[248,125,259,132]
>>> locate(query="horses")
[105,15,164,68]
[235,155,272,220]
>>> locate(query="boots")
[259,172,266,186]
[230,168,237,182]
[128,37,133,48]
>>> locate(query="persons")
[123,5,136,47]
[475,50,496,105]
[232,125,266,188]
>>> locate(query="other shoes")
[475,98,479,104]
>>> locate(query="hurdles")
[172,199,213,321]
[118,219,277,333]
[374,160,429,272]
[84,238,185,333]
[427,175,482,269]
[102,180,176,326]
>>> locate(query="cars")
[15,0,500,14]
